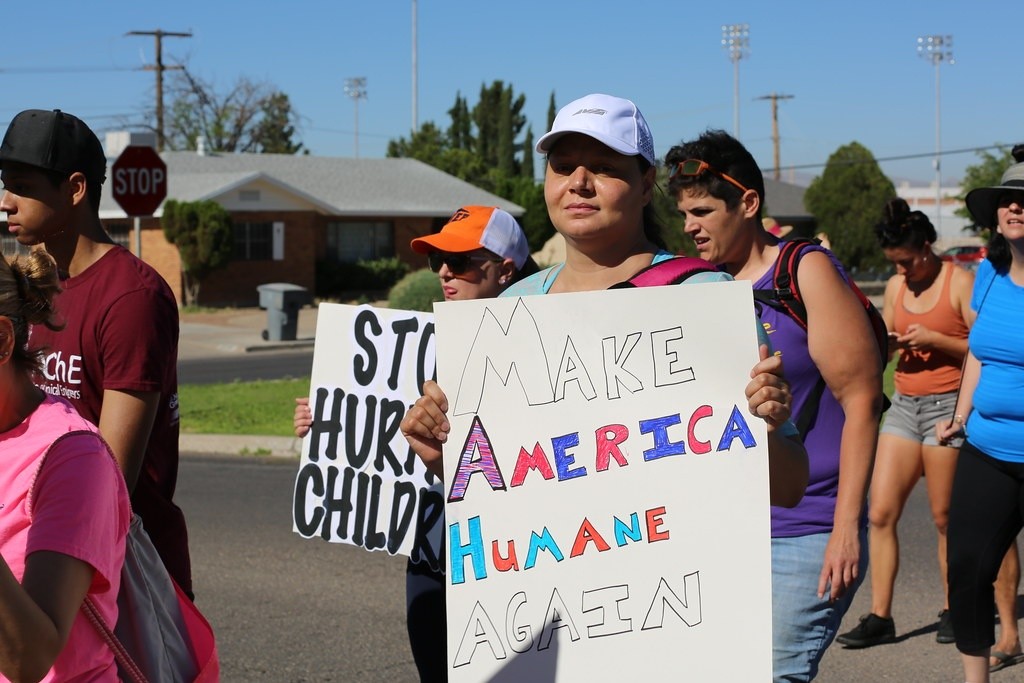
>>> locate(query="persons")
[665,131,884,683]
[295,206,539,683]
[399,95,808,683]
[836,199,980,645]
[937,160,1024,683]
[0,247,127,682]
[0,108,192,604]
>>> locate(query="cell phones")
[887,332,901,340]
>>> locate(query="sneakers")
[936,610,956,643]
[836,615,896,647]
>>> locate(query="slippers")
[988,650,1024,672]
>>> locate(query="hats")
[965,162,1024,230]
[536,94,655,166]
[761,216,793,239]
[411,206,529,270]
[0,109,106,183]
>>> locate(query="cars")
[940,244,991,266]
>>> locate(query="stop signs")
[111,144,169,219]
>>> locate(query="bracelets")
[955,415,963,423]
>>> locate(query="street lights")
[918,33,957,240]
[342,76,372,156]
[718,23,752,145]
[751,90,795,182]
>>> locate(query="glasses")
[427,251,504,275]
[669,159,747,192]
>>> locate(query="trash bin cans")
[258,282,306,342]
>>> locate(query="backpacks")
[26,430,220,683]
